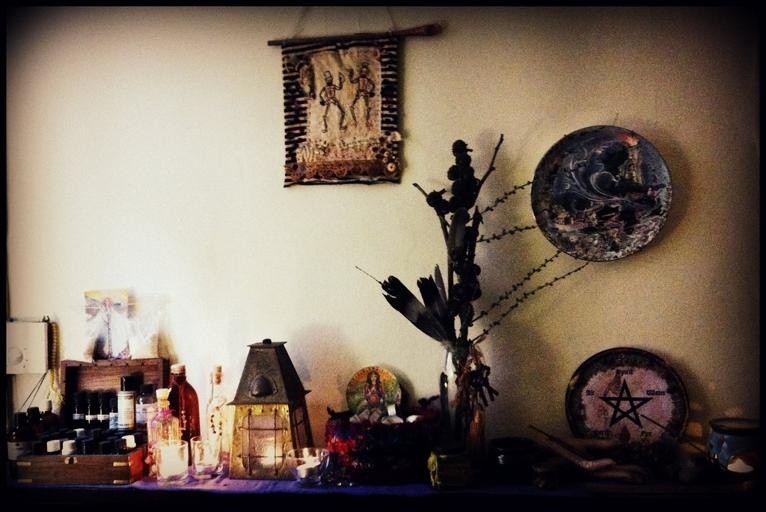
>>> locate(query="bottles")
[6,362,230,480]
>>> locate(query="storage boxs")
[14,357,167,485]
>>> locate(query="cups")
[286,448,330,487]
[155,433,220,487]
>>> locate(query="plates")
[346,367,402,415]
[565,347,690,455]
[531,125,673,262]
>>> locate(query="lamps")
[226,339,313,481]
